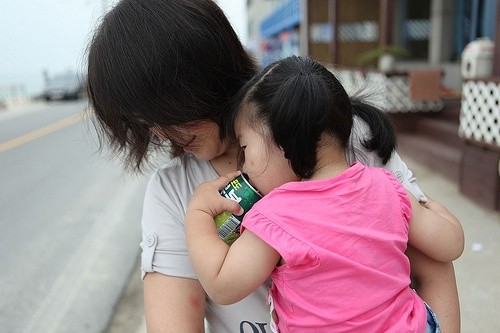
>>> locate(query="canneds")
[213,172,265,244]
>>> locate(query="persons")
[86,0,460,333]
[183,54,465,333]
